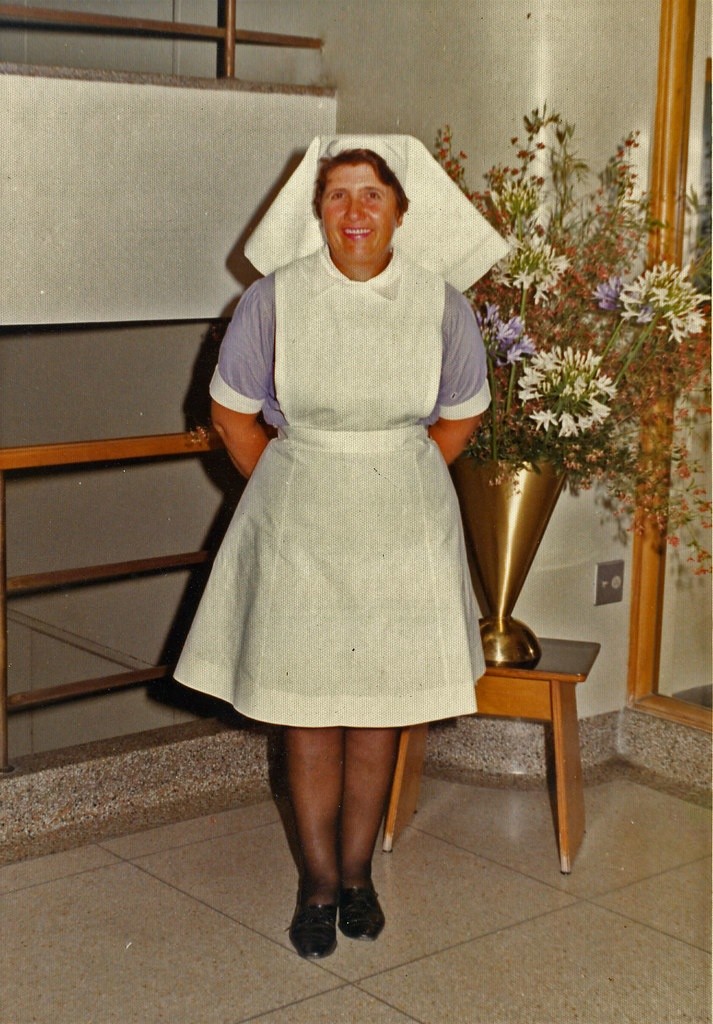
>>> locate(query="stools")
[376,638,602,877]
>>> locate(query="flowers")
[185,101,712,583]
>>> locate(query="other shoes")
[340,886,386,941]
[290,901,340,959]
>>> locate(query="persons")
[198,134,495,961]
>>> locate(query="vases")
[444,459,570,670]
